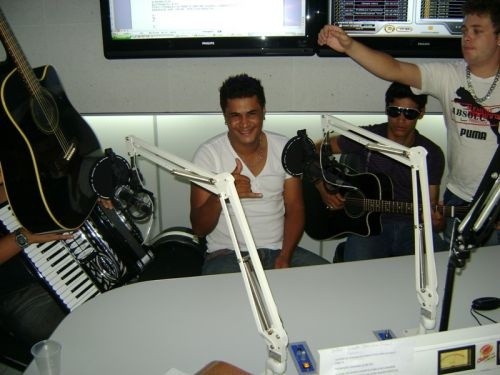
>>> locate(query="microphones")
[113,184,151,215]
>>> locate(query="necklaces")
[465,65,500,102]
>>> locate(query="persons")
[316,0,500,247]
[312,79,451,263]
[0,164,114,341]
[189,74,332,275]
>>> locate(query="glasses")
[385,106,420,120]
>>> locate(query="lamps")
[282,110,441,336]
[88,133,290,375]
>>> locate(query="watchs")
[15,229,29,248]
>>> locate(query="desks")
[25,244,500,375]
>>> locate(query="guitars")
[0,7,105,236]
[302,155,474,242]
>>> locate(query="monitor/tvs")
[100,0,464,60]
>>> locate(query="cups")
[30,340,61,375]
